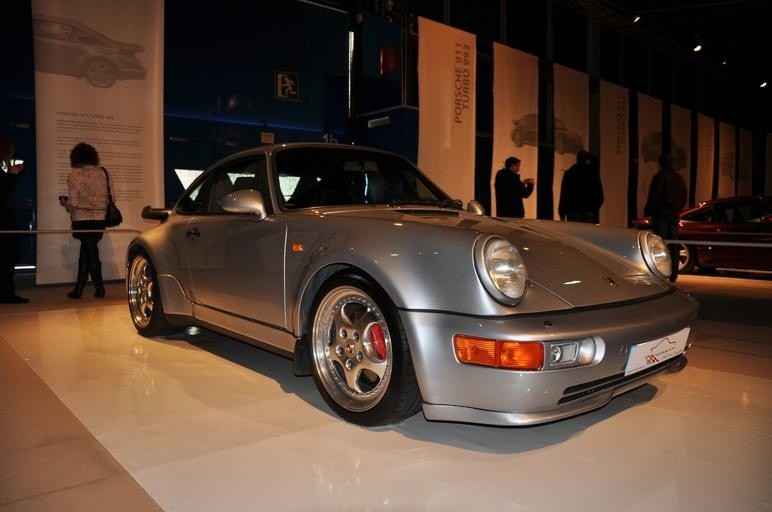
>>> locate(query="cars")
[721,151,749,183]
[125,142,700,427]
[511,114,584,155]
[32,14,147,88]
[641,131,688,171]
[632,195,772,274]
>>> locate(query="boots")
[68,259,105,298]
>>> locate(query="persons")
[494,156,535,218]
[60,143,116,297]
[643,154,687,282]
[558,150,604,223]
[0,136,30,304]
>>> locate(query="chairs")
[687,207,746,224]
[195,167,366,214]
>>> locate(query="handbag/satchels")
[102,167,122,226]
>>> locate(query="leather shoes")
[0,295,29,304]
